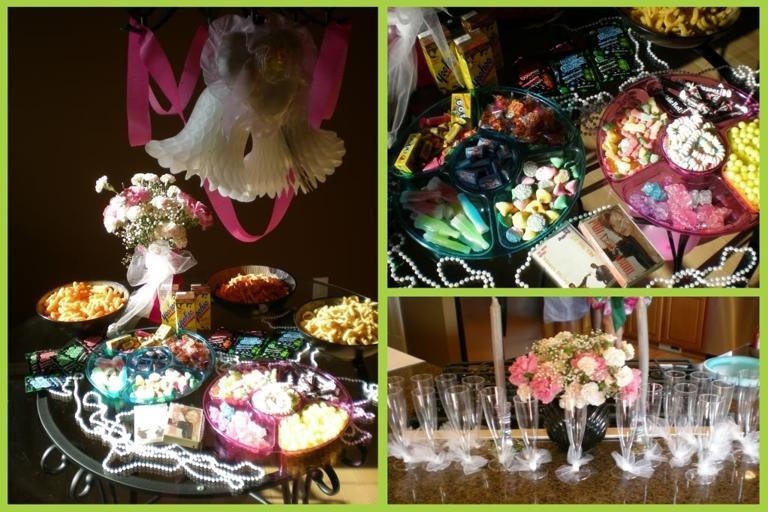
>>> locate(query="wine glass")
[390,370,761,485]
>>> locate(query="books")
[531,204,665,289]
[134,402,204,449]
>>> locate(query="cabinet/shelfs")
[400,297,541,369]
[629,297,755,357]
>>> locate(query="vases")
[544,396,612,452]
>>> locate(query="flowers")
[510,327,643,412]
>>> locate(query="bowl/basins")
[208,265,380,359]
[703,356,759,401]
[37,280,130,331]
